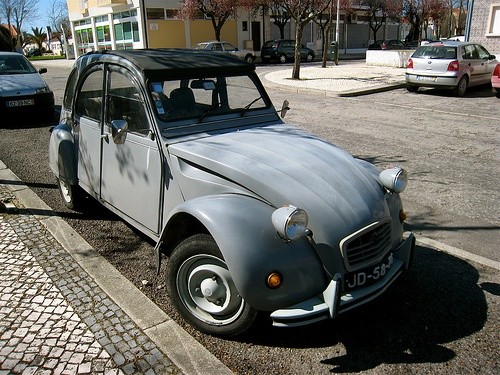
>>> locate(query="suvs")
[261,39,315,63]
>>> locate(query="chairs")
[5,57,19,69]
[166,88,202,119]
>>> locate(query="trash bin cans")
[330,41,338,61]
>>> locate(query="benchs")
[80,91,161,126]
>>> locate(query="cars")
[49,47,416,338]
[491,62,500,98]
[406,41,500,97]
[419,35,465,47]
[195,41,256,64]
[368,39,410,50]
[0,52,56,122]
